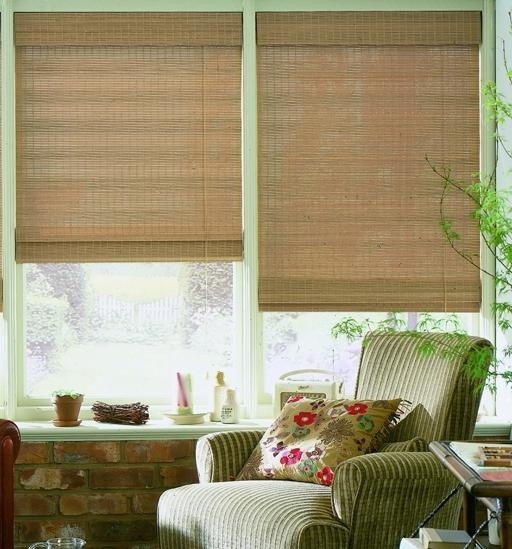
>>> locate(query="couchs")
[155,328,496,549]
[0,417,22,548]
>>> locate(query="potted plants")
[49,387,86,428]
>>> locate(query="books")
[479,447,512,467]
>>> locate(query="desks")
[399,436,512,548]
